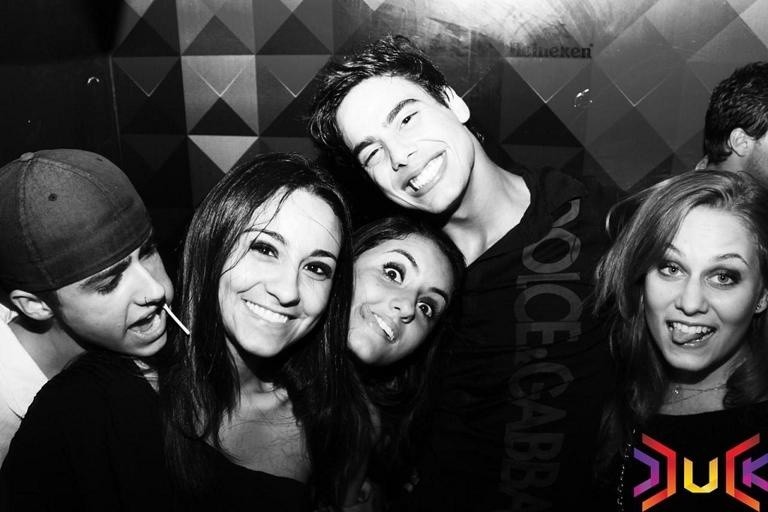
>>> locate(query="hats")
[0,149,152,293]
